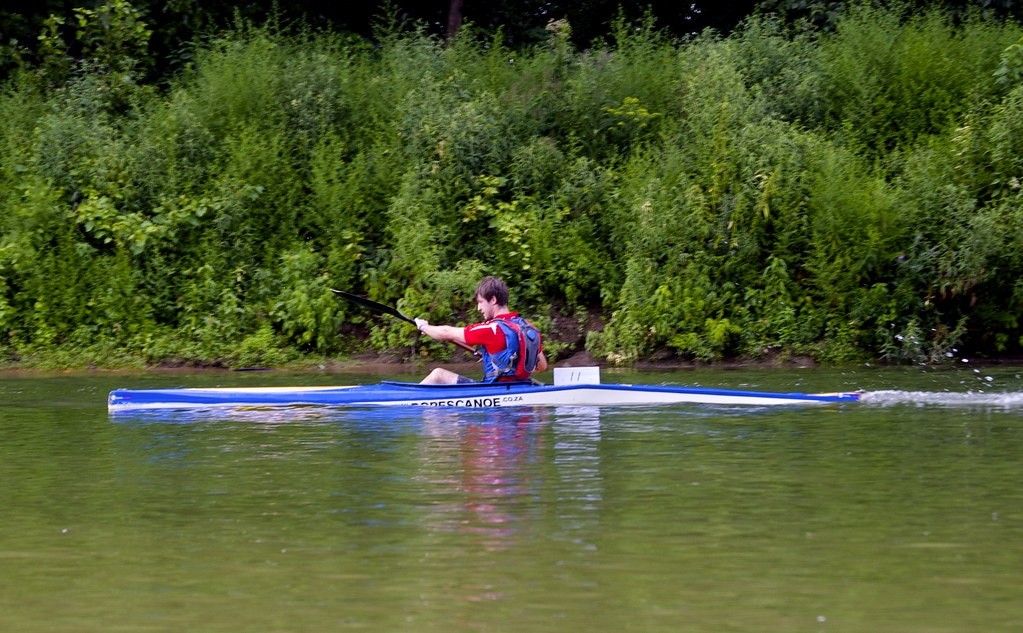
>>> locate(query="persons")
[413,276,548,385]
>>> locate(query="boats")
[107,362,865,414]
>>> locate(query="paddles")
[332,286,542,388]
